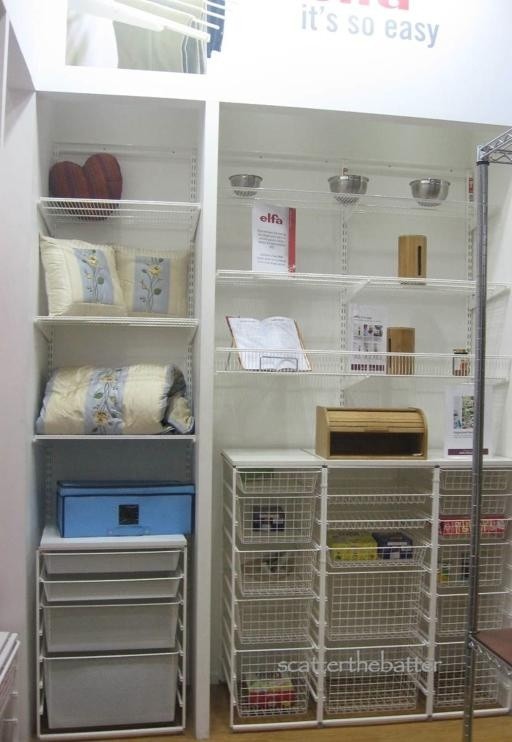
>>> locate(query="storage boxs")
[43,481,193,730]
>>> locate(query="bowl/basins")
[326,175,371,205]
[408,178,452,208]
[228,174,264,197]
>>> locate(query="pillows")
[111,244,192,318]
[38,231,128,316]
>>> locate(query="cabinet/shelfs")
[221,446,511,732]
[217,186,512,382]
[33,196,199,440]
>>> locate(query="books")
[226,316,310,371]
